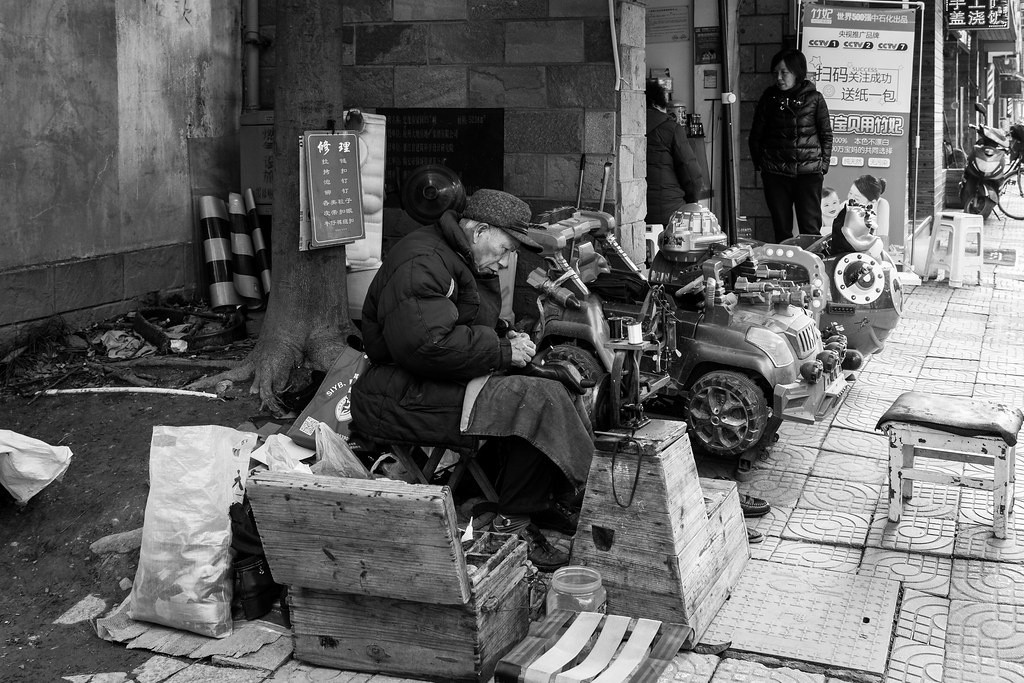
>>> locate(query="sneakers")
[530,490,578,537]
[518,519,572,572]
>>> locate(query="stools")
[494,610,695,683]
[924,225,984,287]
[874,393,1024,539]
[923,212,984,282]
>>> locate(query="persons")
[647,79,703,230]
[943,138,968,168]
[748,48,832,243]
[1009,116,1024,174]
[819,175,889,253]
[350,189,596,572]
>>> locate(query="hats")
[461,189,544,257]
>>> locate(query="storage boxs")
[244,471,536,683]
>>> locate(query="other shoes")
[739,492,772,517]
[747,525,764,543]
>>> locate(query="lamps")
[1002,55,1010,66]
